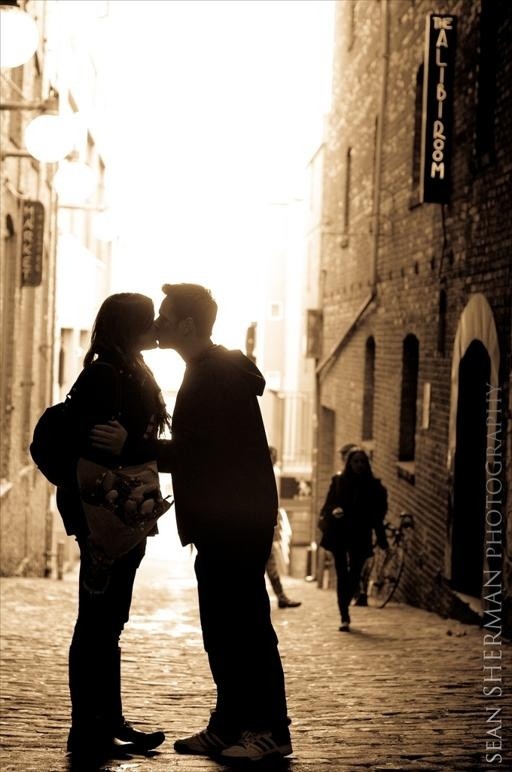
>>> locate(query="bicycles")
[360,509,415,609]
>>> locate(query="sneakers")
[174,726,292,761]
[279,600,301,607]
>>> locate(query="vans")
[271,458,313,549]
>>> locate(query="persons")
[318,446,392,633]
[265,444,303,609]
[85,283,282,767]
[339,442,390,607]
[25,291,169,762]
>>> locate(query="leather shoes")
[67,717,165,752]
[340,627,349,631]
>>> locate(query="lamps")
[0,83,79,166]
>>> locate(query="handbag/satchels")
[30,362,118,487]
